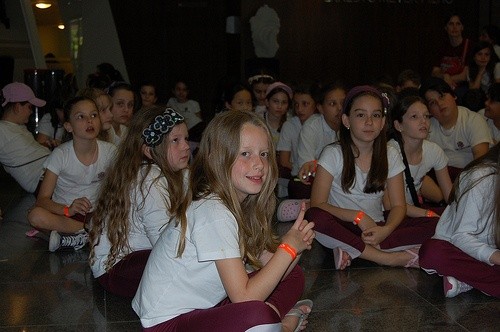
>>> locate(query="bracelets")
[427,210,433,217]
[353,211,365,224]
[314,161,317,171]
[277,243,297,260]
[64,206,69,218]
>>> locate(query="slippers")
[404,247,421,268]
[333,247,351,270]
[285,299,314,332]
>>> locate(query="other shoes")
[277,199,311,221]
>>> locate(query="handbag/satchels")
[441,56,464,75]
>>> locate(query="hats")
[266,82,294,101]
[2,82,46,106]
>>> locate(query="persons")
[130,110,316,331]
[418,141,500,297]
[396,11,500,184]
[87,105,192,295]
[0,83,53,223]
[278,85,347,221]
[303,85,440,269]
[215,72,320,198]
[383,87,453,218]
[34,53,203,151]
[26,97,118,252]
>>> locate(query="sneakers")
[49,230,89,252]
[444,275,473,298]
